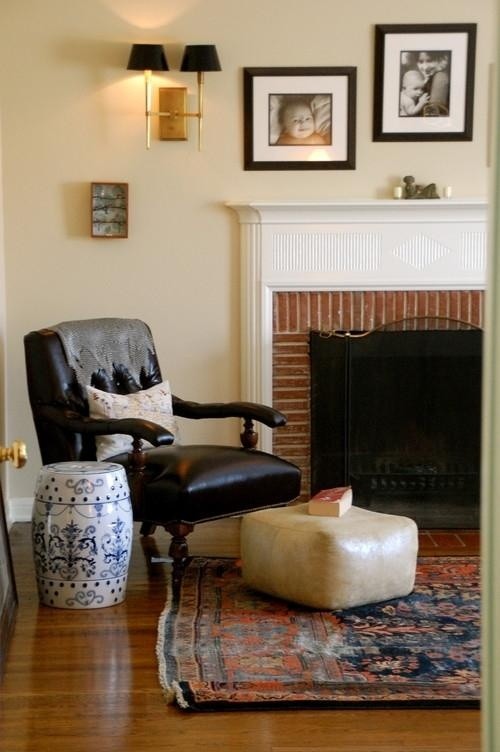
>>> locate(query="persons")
[417,49,449,116]
[400,70,429,115]
[275,99,326,145]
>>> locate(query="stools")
[240,500,418,611]
[32,462,133,609]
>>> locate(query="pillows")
[86,380,184,462]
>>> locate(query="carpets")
[156,546,483,710]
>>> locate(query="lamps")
[179,44,222,151]
[126,44,170,149]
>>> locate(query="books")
[307,486,352,517]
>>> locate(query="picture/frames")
[372,23,475,142]
[243,66,357,170]
[91,182,129,239]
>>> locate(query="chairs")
[24,318,303,591]
